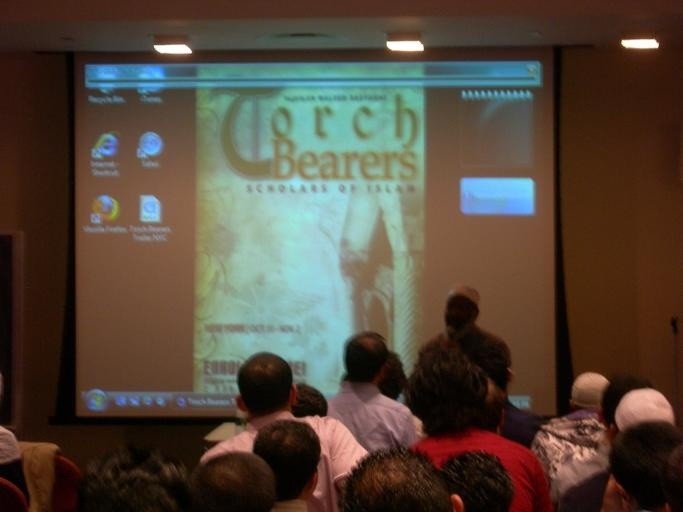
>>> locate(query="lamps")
[386,32,424,52]
[154,35,193,56]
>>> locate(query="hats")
[614,387,675,432]
[570,372,610,408]
[448,287,480,305]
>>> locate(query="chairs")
[9,452,81,512]
[0,477,27,511]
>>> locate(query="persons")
[1,332,682,510]
[403,285,515,434]
